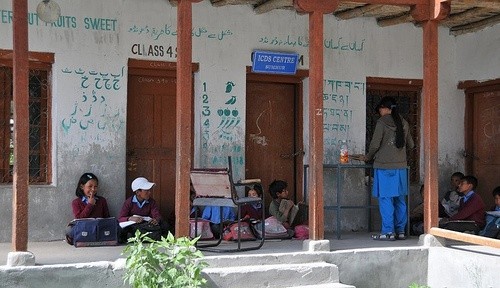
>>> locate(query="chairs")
[190,156,265,253]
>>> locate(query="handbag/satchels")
[189,218,214,240]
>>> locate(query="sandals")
[370,233,408,241]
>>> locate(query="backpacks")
[223,217,309,240]
[121,222,160,241]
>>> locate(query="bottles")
[340,140,349,164]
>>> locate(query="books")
[278,199,308,226]
[119,215,152,229]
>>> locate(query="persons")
[190,189,202,218]
[268,179,294,223]
[71,173,110,219]
[409,172,500,239]
[359,95,415,241]
[116,177,163,245]
[202,206,237,240]
[240,183,269,220]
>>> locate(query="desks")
[304,164,411,240]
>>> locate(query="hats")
[131,177,156,192]
